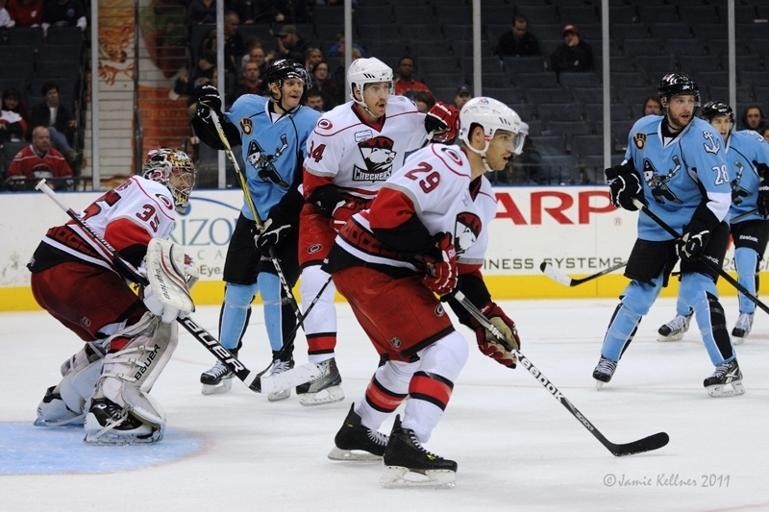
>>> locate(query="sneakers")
[83,400,154,436]
[39,387,85,425]
[297,359,341,394]
[267,360,294,389]
[384,413,457,471]
[704,358,742,386]
[659,315,689,337]
[732,312,753,337]
[336,403,388,456]
[593,356,616,382]
[201,359,234,385]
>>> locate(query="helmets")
[656,72,698,95]
[263,59,312,90]
[347,57,393,107]
[457,97,529,153]
[142,149,196,213]
[702,101,734,124]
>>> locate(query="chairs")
[0,0,95,190]
[404,1,768,184]
[152,1,408,164]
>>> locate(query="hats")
[456,85,471,95]
[563,26,576,34]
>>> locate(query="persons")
[589,70,749,388]
[1,0,768,194]
[28,148,200,444]
[293,57,432,405]
[658,96,769,344]
[322,96,533,482]
[190,59,325,405]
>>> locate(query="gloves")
[605,158,643,210]
[425,101,458,143]
[199,86,221,124]
[469,304,520,367]
[329,200,366,233]
[676,223,710,263]
[422,231,459,296]
[757,186,769,218]
[254,216,293,249]
